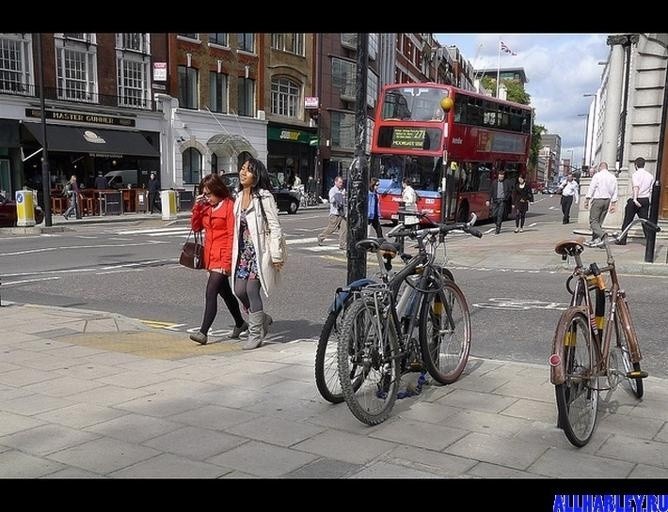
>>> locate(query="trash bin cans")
[160,190,177,221]
[15,191,36,226]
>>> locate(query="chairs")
[47,186,149,216]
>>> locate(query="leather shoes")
[228,320,248,338]
[190,332,207,345]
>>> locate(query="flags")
[501,41,516,56]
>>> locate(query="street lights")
[583,93,597,169]
[577,113,589,166]
[567,149,575,167]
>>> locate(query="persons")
[557,174,578,224]
[190,174,248,345]
[62,174,83,220]
[145,173,161,214]
[446,166,466,191]
[318,177,348,250]
[231,159,285,350]
[511,175,533,233]
[486,170,512,234]
[268,169,322,207]
[367,177,385,245]
[94,170,108,189]
[392,178,424,248]
[583,163,618,248]
[219,169,225,175]
[614,158,654,245]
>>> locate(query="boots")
[243,307,273,349]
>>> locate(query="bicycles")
[547,219,663,450]
[316,209,487,426]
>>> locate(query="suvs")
[220,172,301,216]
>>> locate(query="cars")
[536,180,564,195]
[0,194,44,228]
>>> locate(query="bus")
[366,81,536,223]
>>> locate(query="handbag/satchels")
[269,233,288,270]
[180,242,204,269]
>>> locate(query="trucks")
[103,169,157,190]
